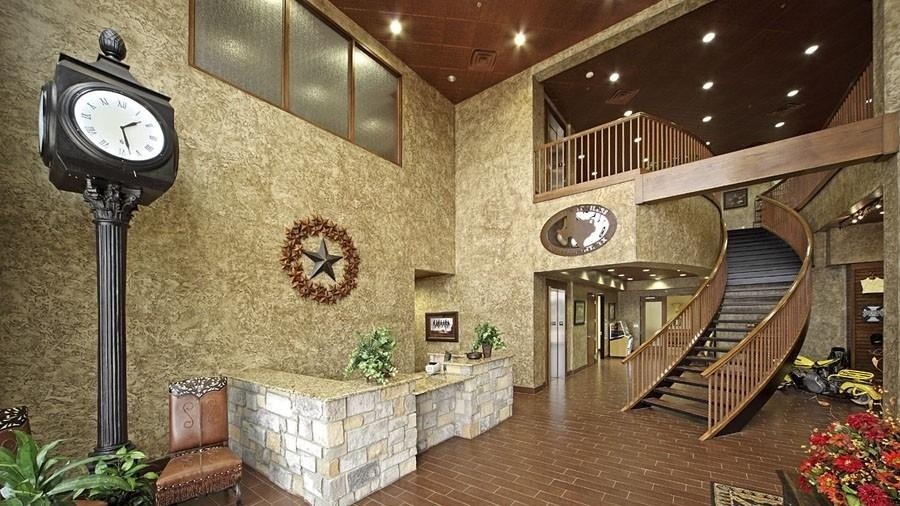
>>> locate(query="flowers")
[799,411,900,505]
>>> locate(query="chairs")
[0,407,109,506]
[154,375,243,505]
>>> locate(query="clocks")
[38,24,179,478]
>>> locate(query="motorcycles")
[778,355,883,407]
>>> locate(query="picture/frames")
[607,302,615,320]
[425,310,459,342]
[573,300,586,325]
[723,188,748,209]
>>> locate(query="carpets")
[710,481,783,505]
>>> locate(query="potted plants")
[347,327,399,384]
[471,323,506,358]
